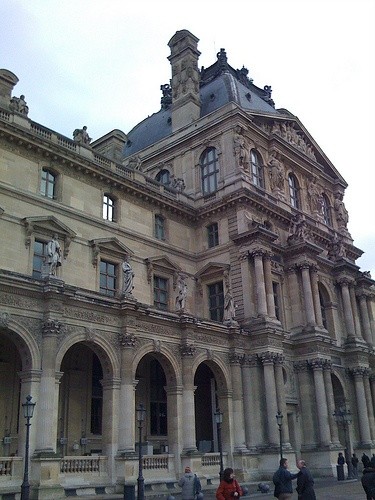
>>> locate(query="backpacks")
[194,473,201,495]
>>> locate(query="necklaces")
[337,452,345,465]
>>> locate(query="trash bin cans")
[123,484,134,499]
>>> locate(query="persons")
[224,286,235,320]
[295,459,317,500]
[307,177,324,214]
[216,468,243,500]
[178,467,201,500]
[326,233,346,260]
[128,153,143,171]
[361,461,375,500]
[288,212,306,238]
[73,126,93,145]
[361,452,375,469]
[47,234,62,277]
[122,256,135,296]
[10,95,29,116]
[272,458,303,500]
[270,119,316,159]
[351,453,358,477]
[267,152,285,191]
[333,193,349,226]
[233,127,250,170]
[174,275,188,309]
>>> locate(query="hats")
[185,467,191,472]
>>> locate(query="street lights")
[21,394,37,500]
[136,403,149,500]
[275,411,284,460]
[332,400,355,479]
[214,408,225,482]
